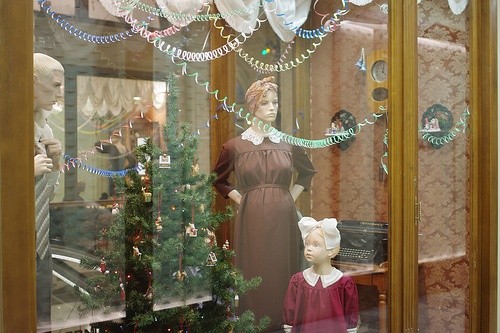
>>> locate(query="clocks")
[366,49,389,115]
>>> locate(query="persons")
[210,76,362,333]
[95,130,137,196]
[33,51,63,332]
